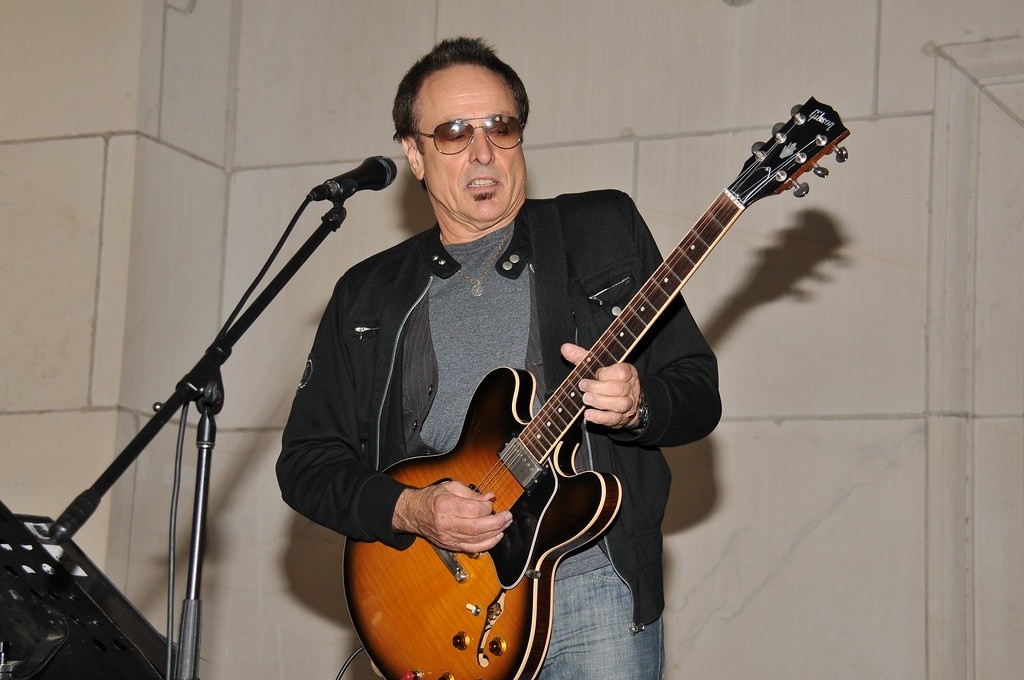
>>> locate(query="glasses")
[405,114,524,155]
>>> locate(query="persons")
[275,32,722,680]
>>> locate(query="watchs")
[622,391,649,432]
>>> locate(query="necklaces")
[456,221,512,296]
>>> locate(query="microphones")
[309,156,397,201]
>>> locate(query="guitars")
[340,95,854,680]
